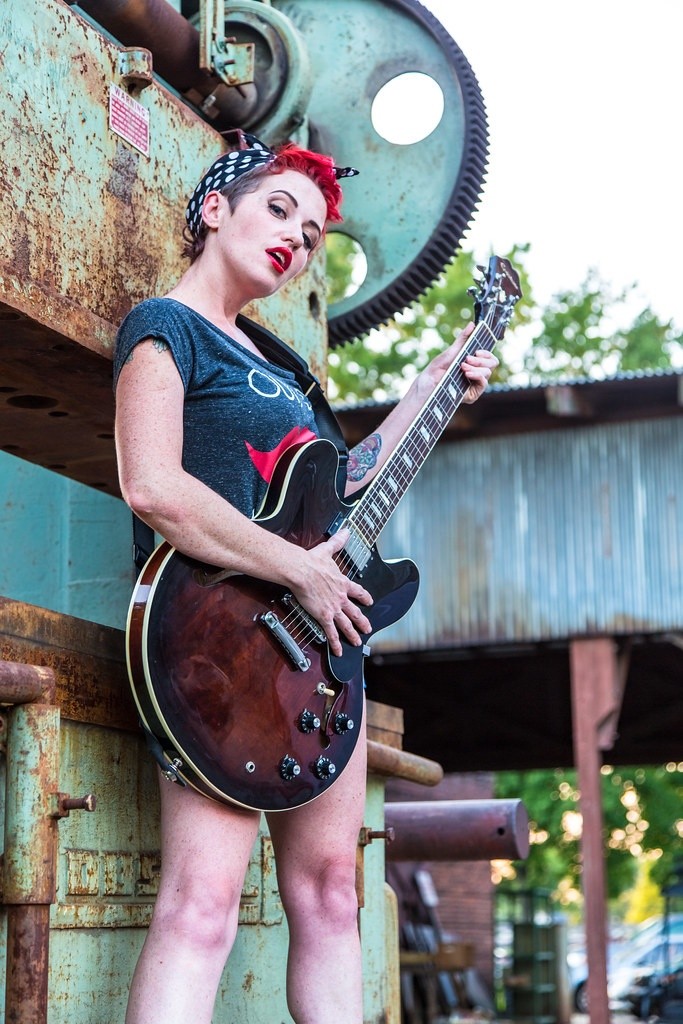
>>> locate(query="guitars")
[125,256,522,813]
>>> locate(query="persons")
[114,133,500,1024]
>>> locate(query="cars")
[565,915,683,1012]
